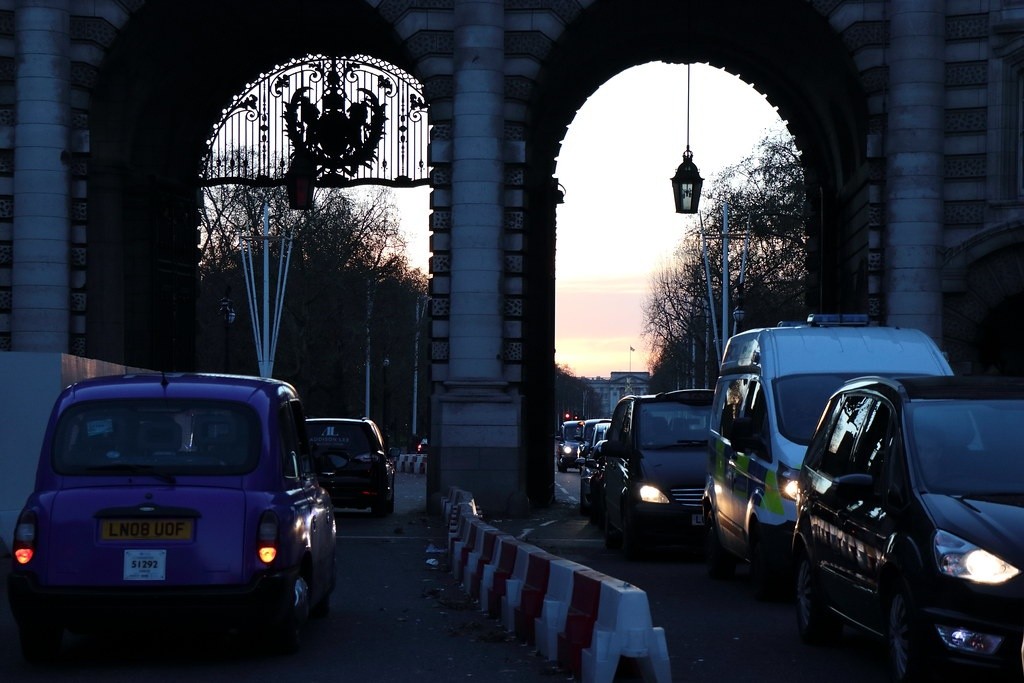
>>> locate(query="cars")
[388,446,405,466]
[555,421,584,473]
[10,373,332,632]
[579,440,610,510]
[417,439,428,454]
[303,417,401,521]
[574,419,611,475]
[600,389,716,549]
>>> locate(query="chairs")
[921,405,975,465]
[839,373,866,386]
[75,410,141,469]
[647,417,668,434]
[245,416,259,451]
[976,403,1024,450]
[193,414,249,459]
[668,417,690,432]
[780,383,831,434]
[138,410,182,458]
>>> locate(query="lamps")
[670,63,705,213]
[283,51,318,210]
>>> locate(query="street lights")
[382,352,388,456]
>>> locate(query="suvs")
[788,374,1024,683]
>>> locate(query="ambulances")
[700,314,989,596]
